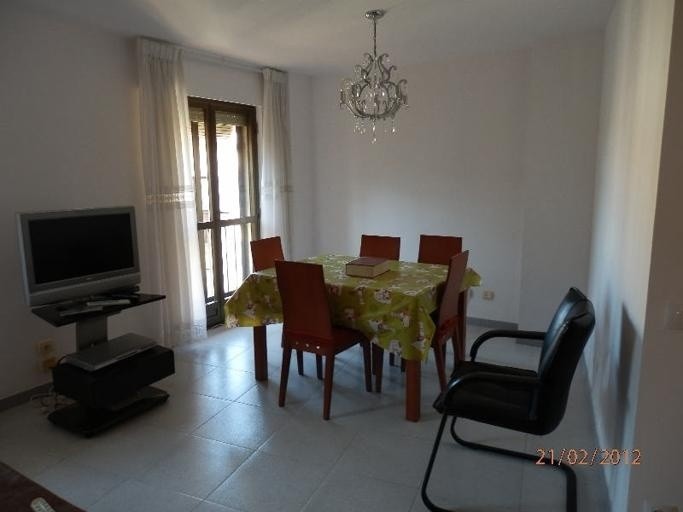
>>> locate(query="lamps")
[337,10,409,144]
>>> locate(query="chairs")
[428,236,469,390]
[417,235,461,374]
[361,235,399,260]
[372,250,469,393]
[273,259,371,420]
[249,237,304,377]
[419,288,594,509]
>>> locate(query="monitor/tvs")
[16,206,141,306]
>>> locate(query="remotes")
[87,299,130,306]
[59,306,103,317]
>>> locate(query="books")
[343,255,393,280]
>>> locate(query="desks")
[228,255,481,420]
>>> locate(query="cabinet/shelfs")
[32,293,175,438]
